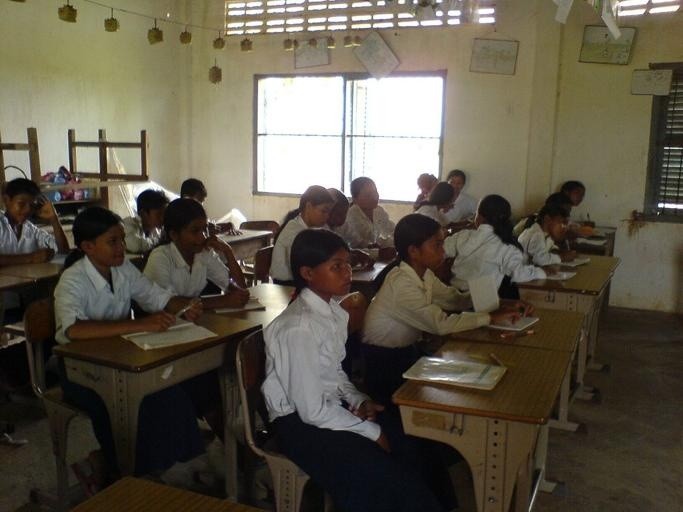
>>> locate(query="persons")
[413,182,475,236]
[258,227,459,511]
[444,168,475,223]
[267,185,375,286]
[346,176,396,246]
[180,177,244,236]
[322,187,396,261]
[52,204,205,497]
[119,189,169,253]
[441,193,558,296]
[511,179,595,268]
[140,196,250,444]
[360,211,534,490]
[0,177,68,267]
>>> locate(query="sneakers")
[192,471,225,492]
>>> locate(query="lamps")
[207,57,221,85]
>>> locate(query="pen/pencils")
[490,352,511,373]
[229,278,246,291]
[45,242,50,249]
[565,239,570,251]
[500,329,536,338]
[175,302,194,318]
[587,213,591,221]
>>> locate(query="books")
[400,356,507,392]
[119,315,219,352]
[485,315,538,332]
[200,291,266,314]
